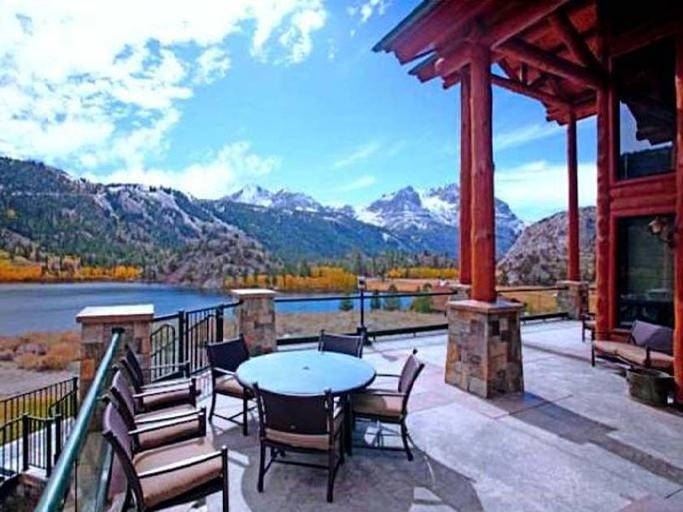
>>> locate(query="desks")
[236,349,376,457]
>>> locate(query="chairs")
[120,344,202,402]
[317,328,365,353]
[352,348,425,462]
[97,394,229,512]
[203,333,264,435]
[582,288,596,341]
[109,362,207,443]
[252,380,351,504]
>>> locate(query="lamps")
[648,216,676,248]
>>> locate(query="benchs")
[591,320,673,375]
